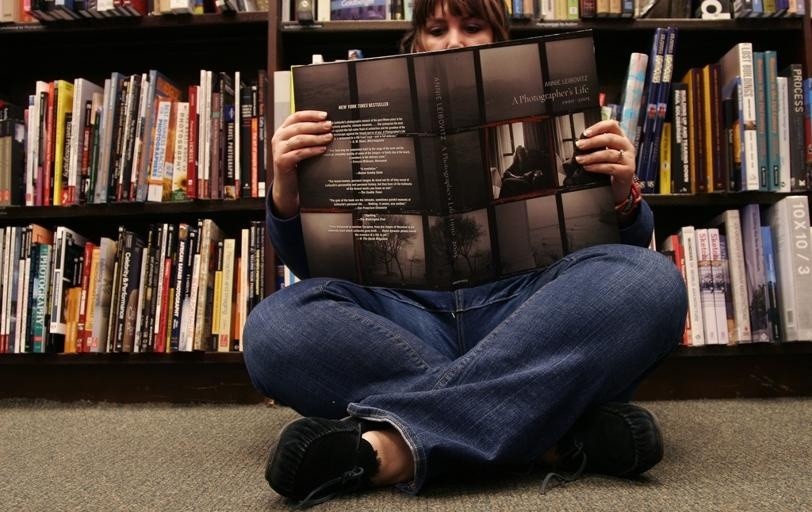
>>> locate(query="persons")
[238,0,689,508]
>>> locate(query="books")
[1,219,265,355]
[282,0,418,23]
[0,0,268,25]
[274,253,301,290]
[313,49,362,64]
[505,0,812,345]
[0,69,267,206]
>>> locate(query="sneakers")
[264,417,397,502]
[556,401,663,479]
